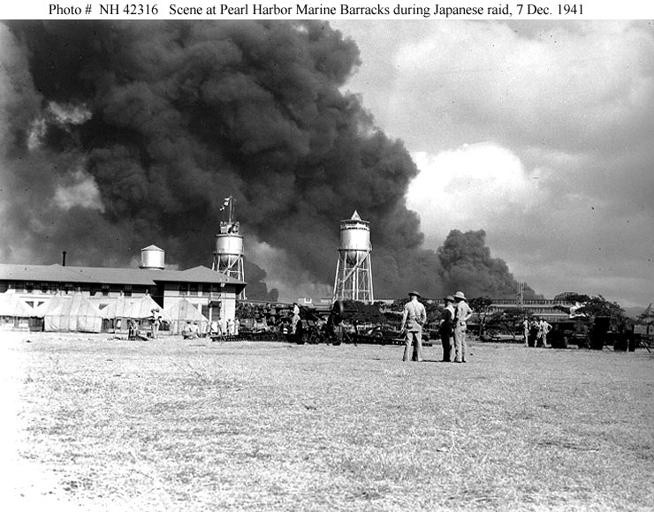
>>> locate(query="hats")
[453,291,467,300]
[408,291,421,298]
[443,296,455,303]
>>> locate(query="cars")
[371,326,401,338]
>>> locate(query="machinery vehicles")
[549,316,636,352]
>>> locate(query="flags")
[220,196,230,212]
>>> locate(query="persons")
[291,303,301,334]
[440,295,455,362]
[453,291,472,363]
[399,291,427,361]
[182,318,239,341]
[521,317,552,348]
